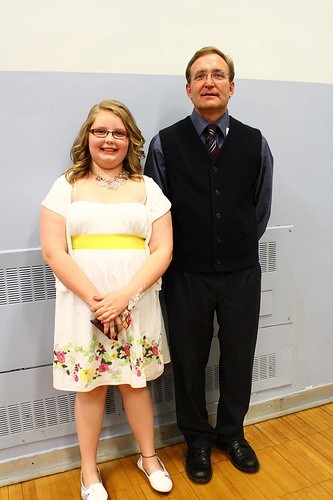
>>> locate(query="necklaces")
[89,167,130,190]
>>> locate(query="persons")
[144,47,274,483]
[40,99,174,500]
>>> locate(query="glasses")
[88,128,129,140]
[188,71,233,82]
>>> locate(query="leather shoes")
[185,445,212,483]
[80,465,108,500]
[221,435,260,472]
[136,454,174,492]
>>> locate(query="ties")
[204,124,220,160]
